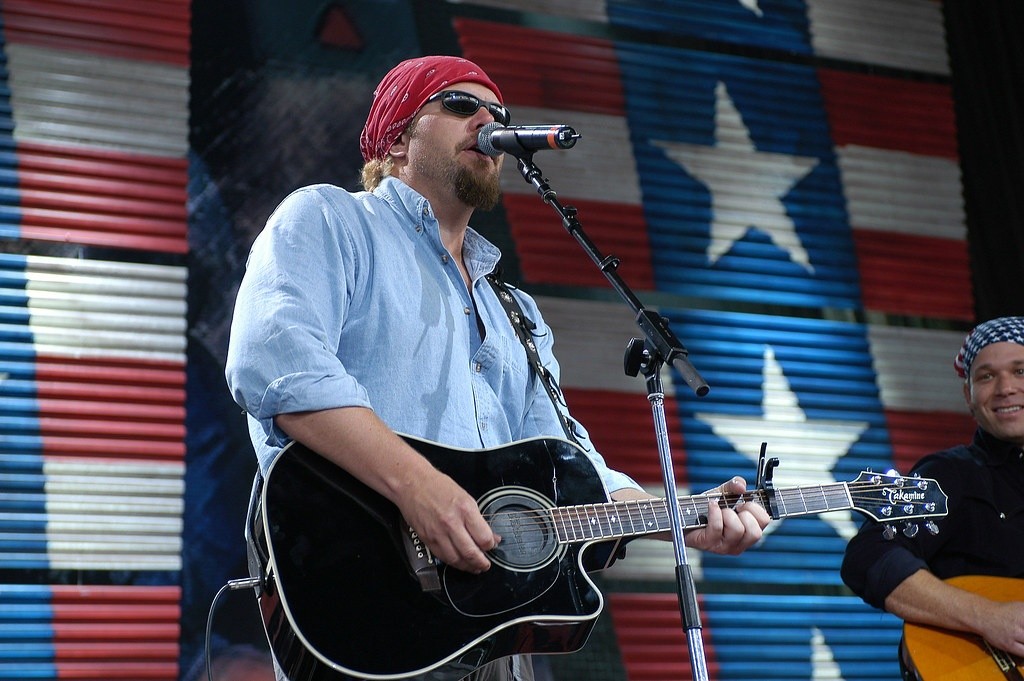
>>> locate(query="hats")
[953,316,1024,379]
[358,53,505,165]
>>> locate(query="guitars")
[897,577,1024,681]
[244,424,950,681]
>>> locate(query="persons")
[840,317,1024,681]
[225,56,771,681]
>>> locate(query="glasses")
[425,89,512,128]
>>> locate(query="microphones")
[478,121,582,157]
